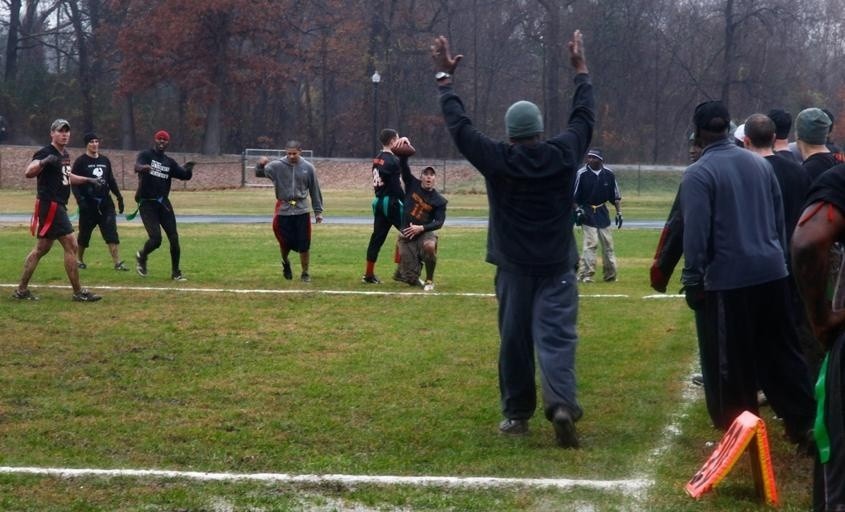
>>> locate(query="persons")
[388,134,449,293]
[254,139,325,280]
[362,128,407,282]
[428,27,597,451]
[132,126,198,281]
[573,141,625,288]
[13,119,106,303]
[70,132,130,274]
[649,99,845,511]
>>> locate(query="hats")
[689,99,834,144]
[587,147,604,161]
[504,100,545,139]
[51,119,71,131]
[154,130,170,141]
[420,166,436,178]
[84,133,100,147]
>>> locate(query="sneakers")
[77,260,86,268]
[281,260,293,280]
[551,405,579,450]
[300,272,312,282]
[392,267,439,291]
[499,419,528,434]
[114,260,130,271]
[12,288,40,300]
[362,273,384,284]
[171,270,187,281]
[136,250,150,277]
[72,288,102,302]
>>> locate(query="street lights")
[371,70,381,157]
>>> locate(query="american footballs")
[393,144,419,158]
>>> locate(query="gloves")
[685,285,707,310]
[575,207,585,226]
[615,212,623,228]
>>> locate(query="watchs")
[435,71,453,83]
